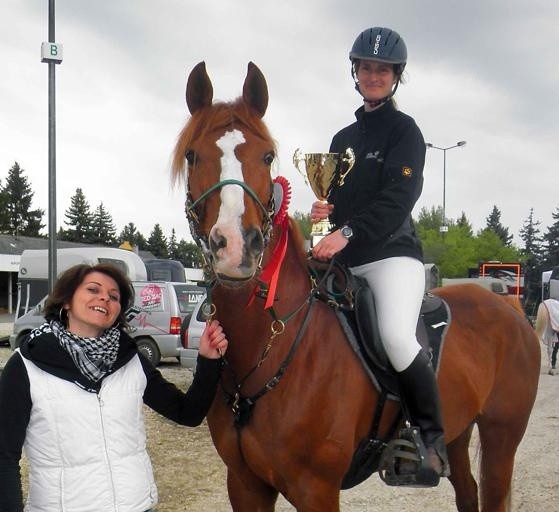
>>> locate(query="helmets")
[349,27,407,64]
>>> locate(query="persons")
[311,27,451,477]
[0,263,228,512]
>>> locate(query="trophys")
[292,145,356,236]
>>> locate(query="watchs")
[339,224,353,244]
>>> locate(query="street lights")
[422,140,466,275]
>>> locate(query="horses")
[169,61,542,512]
[535,298,559,375]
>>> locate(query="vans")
[9,280,210,376]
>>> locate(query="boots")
[394,348,452,476]
